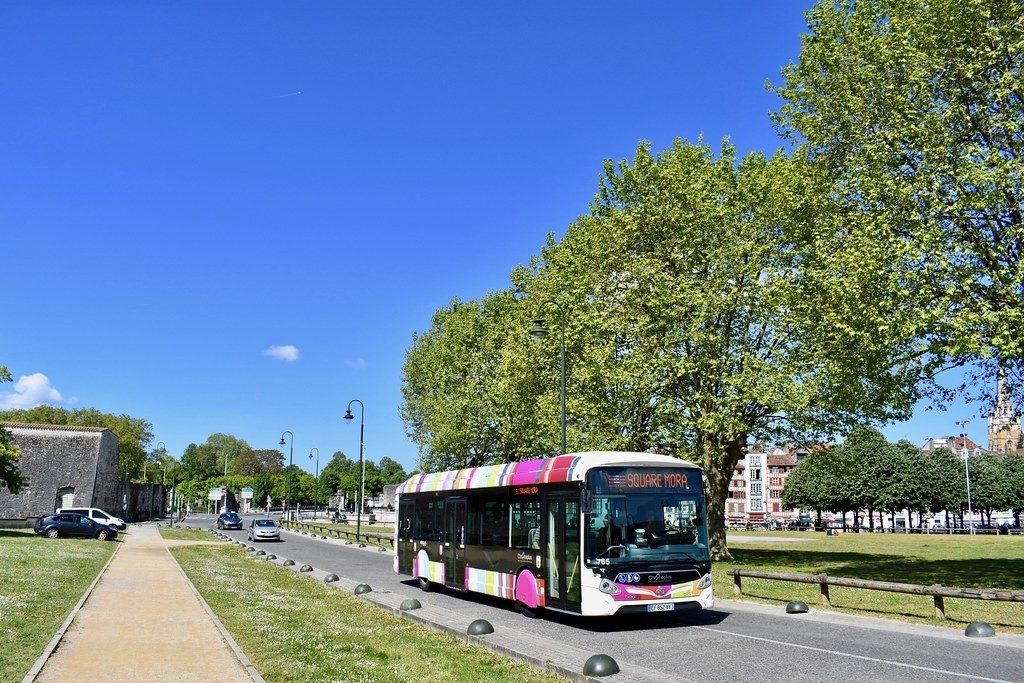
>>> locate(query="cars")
[216,512,243,530]
[724,511,1014,530]
[248,518,281,543]
[34,512,119,541]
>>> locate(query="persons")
[633,506,672,549]
[594,514,622,558]
[533,526,541,549]
[334,509,341,524]
[784,519,827,531]
[256,521,260,526]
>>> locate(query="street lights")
[154,441,168,483]
[279,430,293,528]
[307,448,319,516]
[955,420,974,535]
[528,300,567,455]
[170,462,183,528]
[343,400,364,542]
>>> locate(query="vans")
[56,507,127,530]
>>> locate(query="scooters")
[330,513,348,525]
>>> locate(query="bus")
[392,449,716,619]
